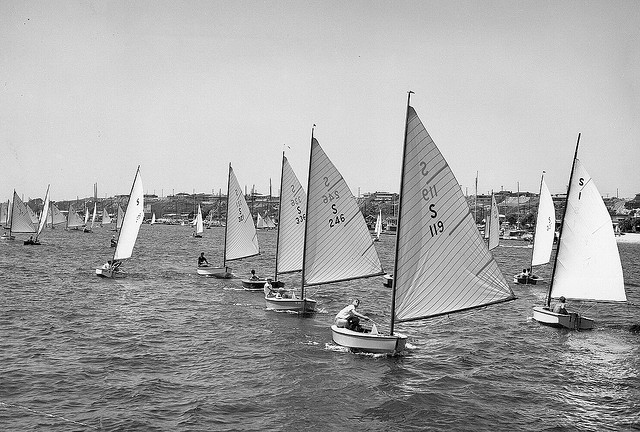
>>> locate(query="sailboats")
[331,91,518,354]
[514,171,555,285]
[96,165,144,278]
[257,213,275,231]
[51,204,67,228]
[111,205,124,232]
[65,205,84,230]
[26,205,38,223]
[83,202,96,233]
[3,199,11,229]
[265,124,387,315]
[195,204,203,237]
[151,213,155,225]
[82,207,89,227]
[24,184,50,245]
[488,189,499,250]
[197,162,259,278]
[1,188,37,240]
[533,134,627,330]
[97,208,111,228]
[242,151,307,290]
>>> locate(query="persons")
[111,235,118,247]
[112,259,120,270]
[553,296,567,315]
[28,234,35,243]
[520,268,528,277]
[197,252,211,268]
[264,278,279,298]
[526,268,531,277]
[249,270,261,281]
[335,299,361,329]
[103,260,111,269]
